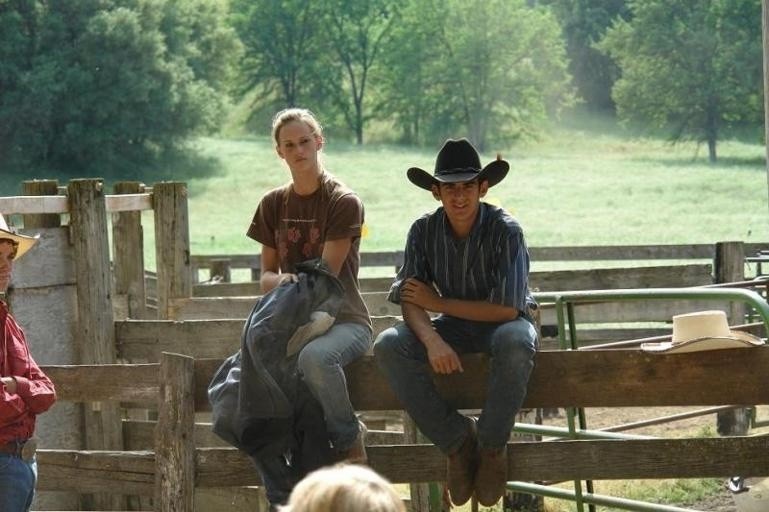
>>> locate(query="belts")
[1,437,42,461]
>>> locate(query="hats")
[406,137,509,191]
[0,210,41,261]
[640,311,765,354]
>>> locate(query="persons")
[0,214,57,511]
[278,460,407,512]
[374,137,540,507]
[210,105,373,512]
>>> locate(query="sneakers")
[336,420,367,466]
[445,416,508,507]
[268,501,286,512]
